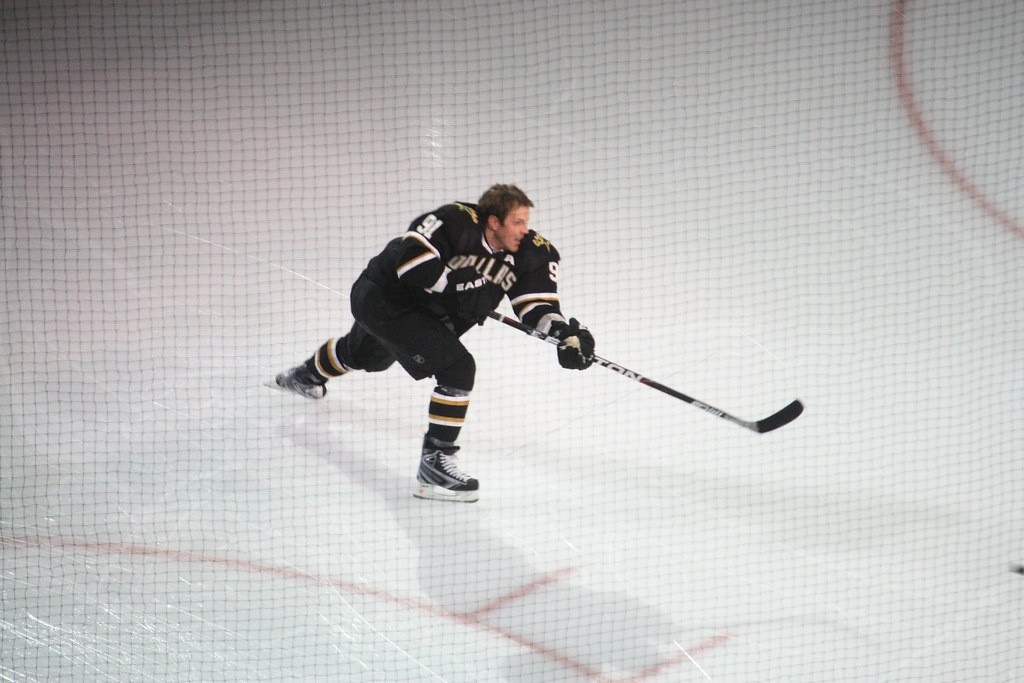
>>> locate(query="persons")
[263,184,594,502]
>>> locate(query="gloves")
[557,317,595,371]
[446,262,489,326]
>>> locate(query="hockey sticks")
[488,310,804,434]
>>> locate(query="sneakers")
[413,436,478,503]
[275,360,327,401]
